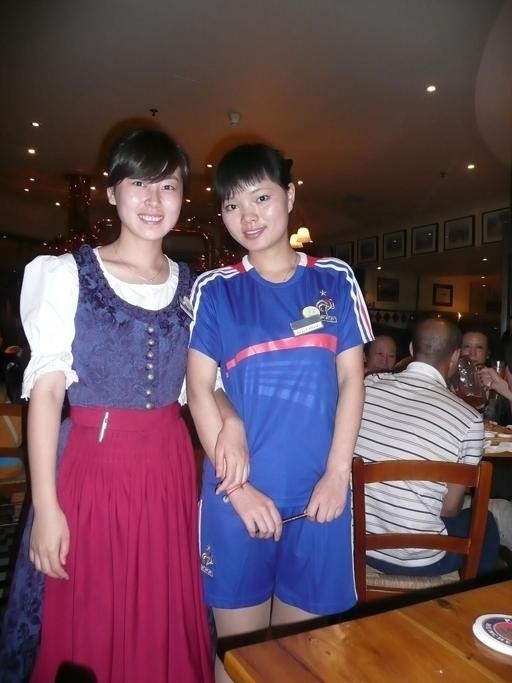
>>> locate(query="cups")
[452,357,487,405]
[491,360,506,379]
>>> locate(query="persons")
[351,318,512,585]
[185,144,374,683]
[20,128,251,683]
[502,362,512,393]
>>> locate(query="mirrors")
[363,245,501,317]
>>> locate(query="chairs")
[352,457,492,605]
[0,403,30,586]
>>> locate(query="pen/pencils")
[255,513,308,534]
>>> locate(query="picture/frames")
[433,284,453,307]
[334,208,503,266]
[377,278,399,301]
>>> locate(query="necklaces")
[112,242,163,283]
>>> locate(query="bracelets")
[223,479,249,503]
